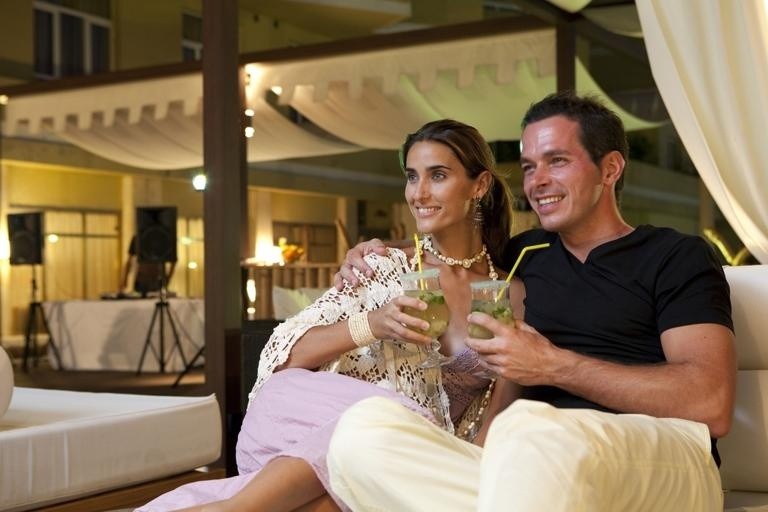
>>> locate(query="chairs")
[713,265,767,512]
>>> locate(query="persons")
[132,119,525,512]
[324,91,737,512]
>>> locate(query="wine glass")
[392,265,454,369]
[467,279,511,377]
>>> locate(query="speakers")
[136,206,176,262]
[7,211,46,264]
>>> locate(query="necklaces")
[411,234,500,440]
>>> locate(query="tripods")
[21,264,63,372]
[136,263,187,375]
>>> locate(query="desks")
[42,298,205,374]
